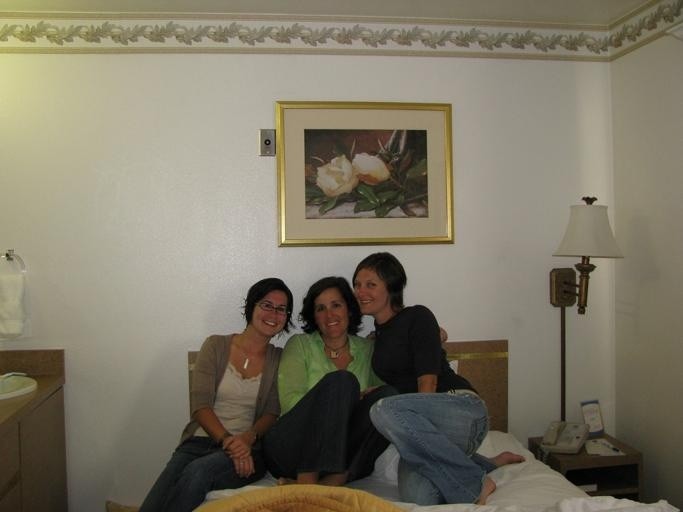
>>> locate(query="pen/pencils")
[602,442,620,452]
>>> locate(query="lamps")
[547,195,626,422]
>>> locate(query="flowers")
[303,129,428,218]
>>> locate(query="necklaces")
[233,340,268,370]
[325,336,349,359]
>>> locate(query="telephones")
[540,421,590,454]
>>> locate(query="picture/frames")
[276,101,452,248]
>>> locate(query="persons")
[352,251,526,505]
[262,276,448,488]
[139,278,295,512]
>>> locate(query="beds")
[185,338,679,512]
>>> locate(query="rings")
[240,462,244,465]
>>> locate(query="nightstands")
[529,430,643,503]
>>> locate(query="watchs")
[248,429,260,444]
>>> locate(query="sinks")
[0,375,38,399]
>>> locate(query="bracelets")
[217,431,232,448]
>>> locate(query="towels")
[0,271,25,342]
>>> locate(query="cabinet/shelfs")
[0,383,71,511]
